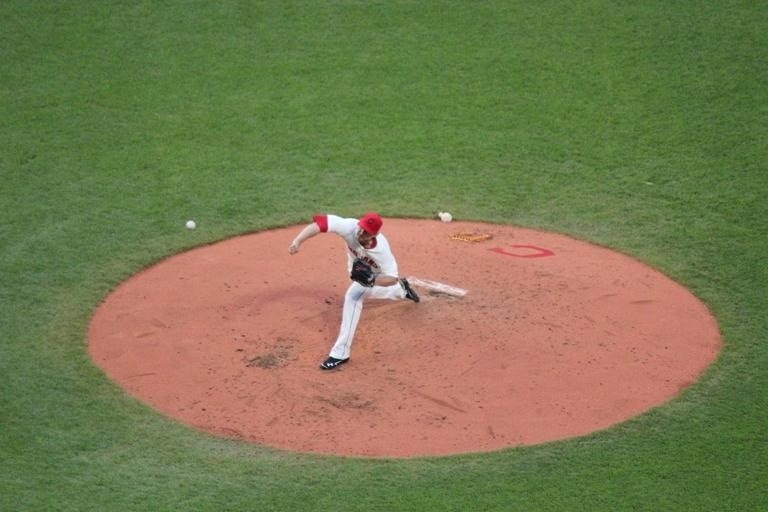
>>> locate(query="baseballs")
[186,220,195,229]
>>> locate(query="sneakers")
[320,356,350,370]
[399,277,420,303]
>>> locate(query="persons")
[288,213,420,370]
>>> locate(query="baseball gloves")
[350,258,375,287]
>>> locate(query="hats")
[358,212,383,236]
